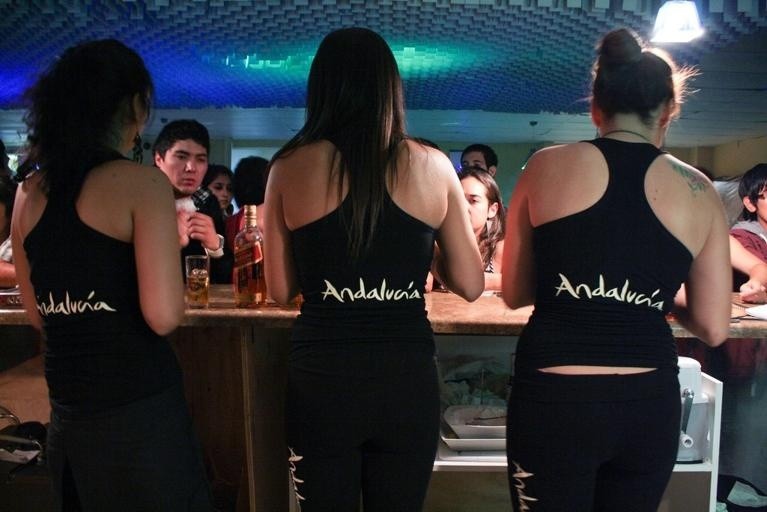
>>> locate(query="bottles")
[233,204,265,306]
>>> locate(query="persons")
[260,26,486,511]
[499,27,734,512]
[8,36,217,512]
[409,137,507,290]
[151,119,271,285]
[0,139,20,186]
[17,135,44,178]
[1,183,37,375]
[726,163,767,306]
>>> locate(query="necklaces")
[601,129,651,141]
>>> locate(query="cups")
[185,254,212,309]
